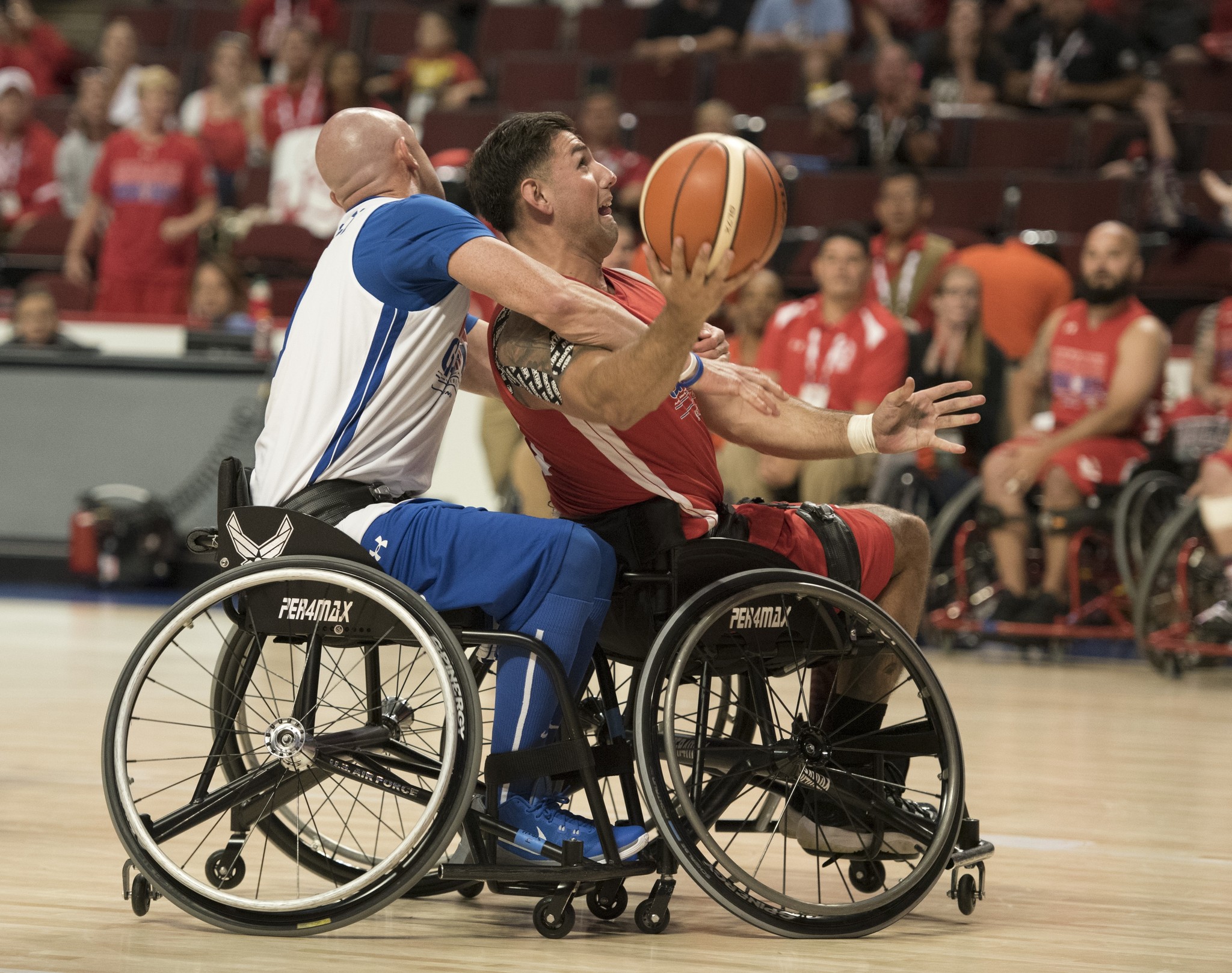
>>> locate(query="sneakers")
[782,792,941,853]
[471,778,650,868]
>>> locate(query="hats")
[0,67,36,99]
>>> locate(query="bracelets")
[678,352,704,388]
[845,412,882,459]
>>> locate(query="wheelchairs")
[103,450,1232,943]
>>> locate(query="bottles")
[67,488,101,585]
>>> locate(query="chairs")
[0,0,1232,323]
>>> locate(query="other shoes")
[1198,599,1231,638]
[993,592,1056,627]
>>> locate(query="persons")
[250,107,793,876]
[0,1,1232,643]
[464,112,987,855]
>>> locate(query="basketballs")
[639,132,787,277]
